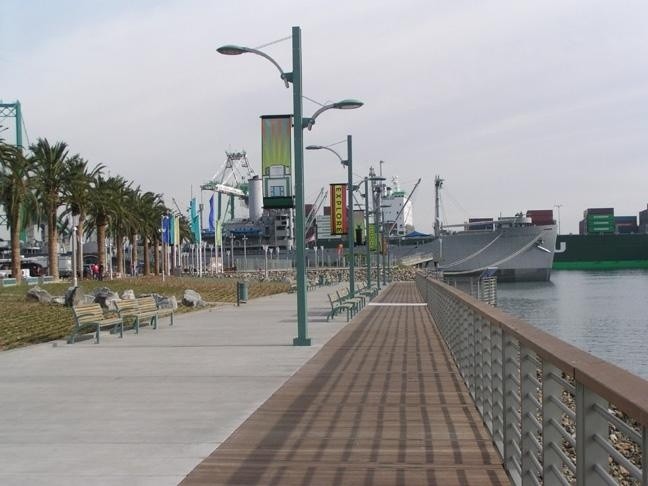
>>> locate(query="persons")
[83,263,104,282]
[22,264,48,277]
[335,244,344,268]
[0,262,13,278]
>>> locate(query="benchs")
[327,281,378,322]
[67,295,174,345]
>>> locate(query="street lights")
[355,176,392,289]
[306,133,387,300]
[215,26,365,345]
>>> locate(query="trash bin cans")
[239,282,248,303]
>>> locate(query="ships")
[124,148,558,281]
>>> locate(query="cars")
[0,253,98,278]
